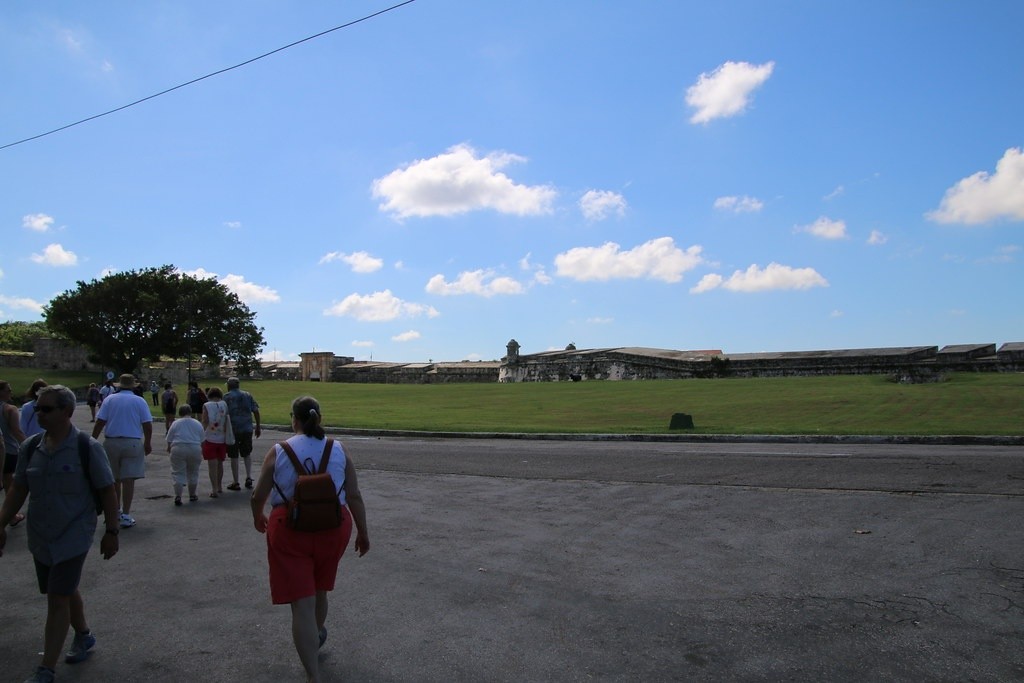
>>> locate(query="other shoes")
[90,419,95,422]
[21,664,56,683]
[65,629,96,663]
[317,626,328,650]
[209,491,217,498]
[218,488,222,493]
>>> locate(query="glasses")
[33,404,65,414]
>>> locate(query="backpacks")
[275,438,343,529]
[164,390,174,413]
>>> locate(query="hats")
[226,377,239,386]
[112,374,140,389]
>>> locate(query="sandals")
[9,514,25,527]
[175,495,183,505]
[190,495,198,501]
[245,477,252,487]
[227,482,241,490]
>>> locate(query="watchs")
[106,528,120,535]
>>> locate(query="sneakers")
[119,515,136,526]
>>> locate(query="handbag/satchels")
[225,415,236,445]
[199,389,209,404]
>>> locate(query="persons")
[202,377,261,498]
[161,383,178,436]
[250,395,371,683]
[186,381,211,421]
[166,404,206,507]
[0,384,119,683]
[0,379,50,527]
[151,381,161,406]
[86,374,153,526]
